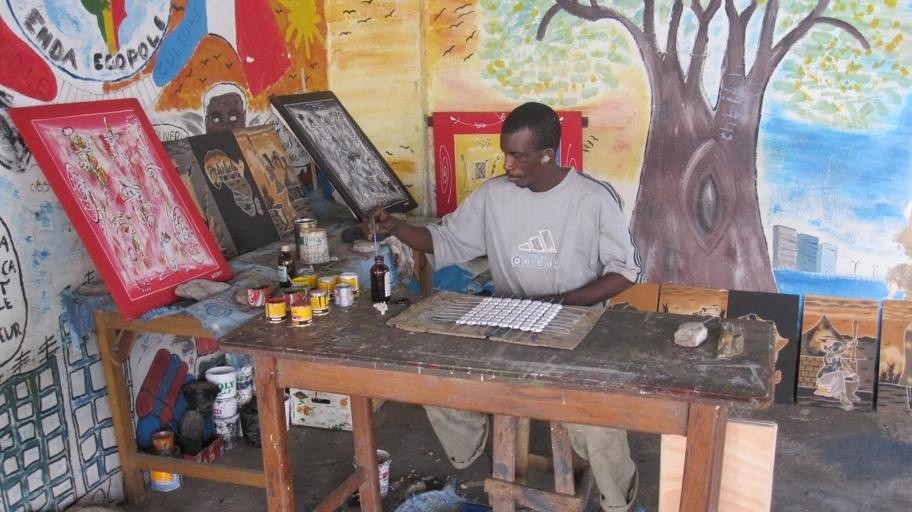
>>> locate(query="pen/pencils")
[371,216,378,257]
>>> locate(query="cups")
[205,366,237,400]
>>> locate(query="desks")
[67,212,444,511]
[218,213,780,512]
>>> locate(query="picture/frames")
[12,97,233,326]
[267,89,419,223]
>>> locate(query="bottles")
[277,244,294,290]
[370,255,391,305]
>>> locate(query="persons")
[203,83,246,134]
[361,102,645,512]
[813,338,861,408]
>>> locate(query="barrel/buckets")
[236,365,254,407]
[207,365,238,422]
[352,449,390,498]
